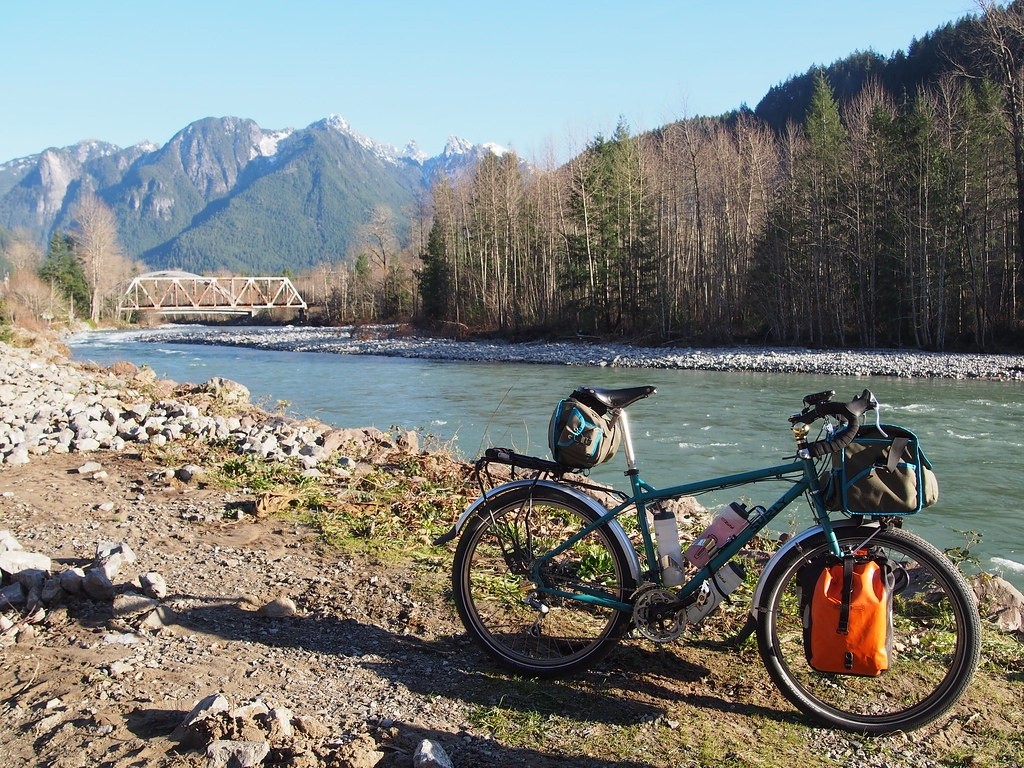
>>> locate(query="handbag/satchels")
[796,547,894,677]
[547,396,622,470]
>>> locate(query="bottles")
[654,509,684,586]
[682,502,749,569]
[687,561,747,623]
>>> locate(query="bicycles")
[432,385,982,735]
